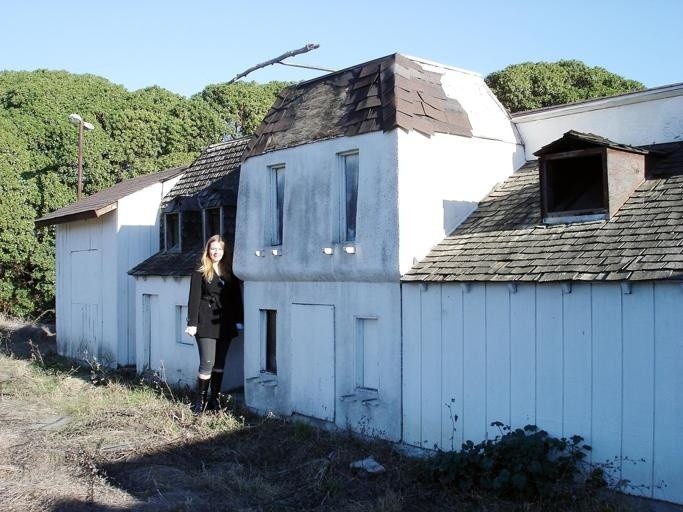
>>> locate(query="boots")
[207,371,233,410]
[192,377,211,416]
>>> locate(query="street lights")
[66,113,94,200]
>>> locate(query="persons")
[184,234,244,414]
